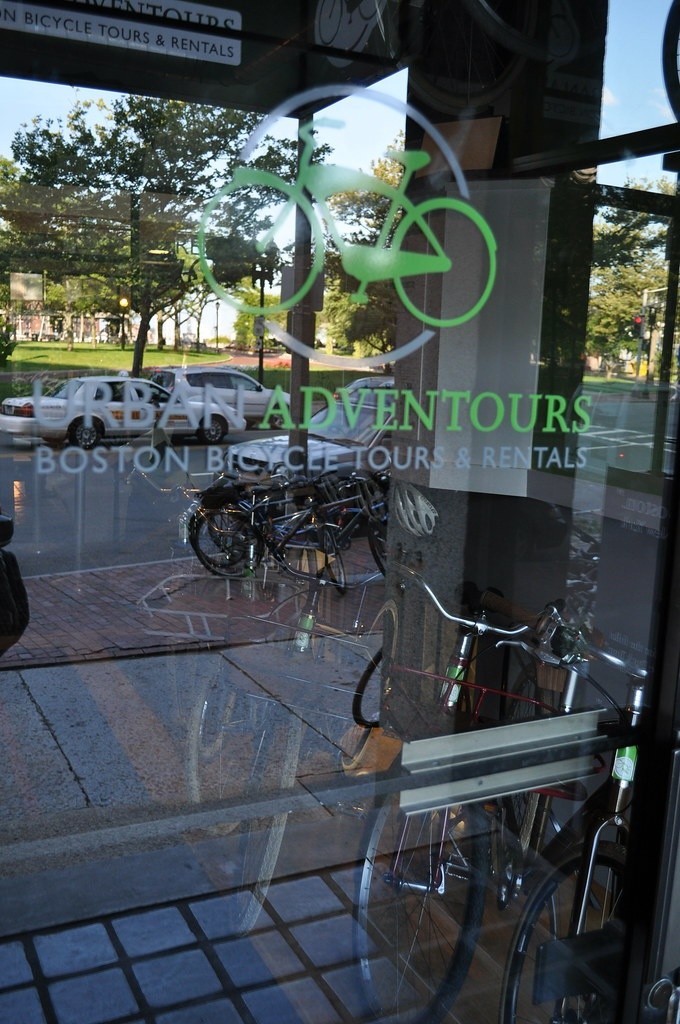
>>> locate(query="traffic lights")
[632,315,643,339]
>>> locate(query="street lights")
[118,298,129,350]
[216,302,220,350]
[247,238,279,386]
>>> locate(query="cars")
[147,365,298,427]
[1,373,248,449]
[334,373,398,404]
[223,393,407,482]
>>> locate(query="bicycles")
[497,597,646,1024]
[223,532,386,939]
[130,485,303,840]
[186,469,387,594]
[350,562,598,1023]
